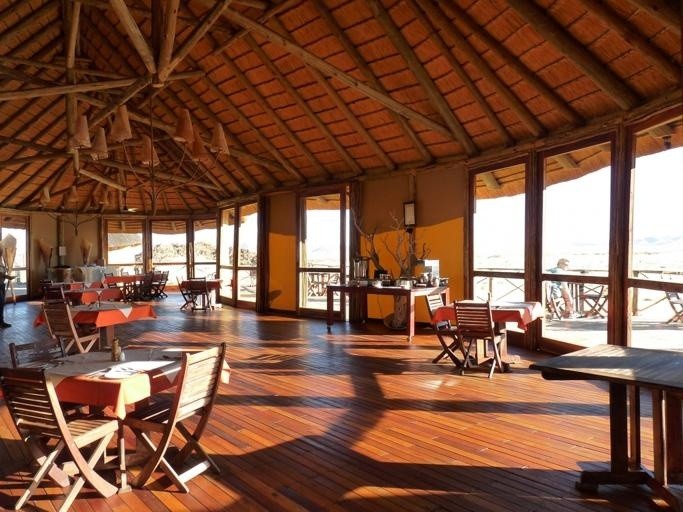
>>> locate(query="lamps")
[33,81,233,238]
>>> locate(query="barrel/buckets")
[45,266,72,283]
[353,257,369,279]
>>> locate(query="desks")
[525,335,683,510]
[319,281,450,343]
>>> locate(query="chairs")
[533,270,683,329]
[38,268,222,353]
[0,337,227,511]
[425,289,542,380]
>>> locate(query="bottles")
[418,272,440,288]
[110,338,126,362]
[89,295,101,309]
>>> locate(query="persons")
[0,232,19,329]
[546,257,574,319]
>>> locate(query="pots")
[354,256,370,278]
